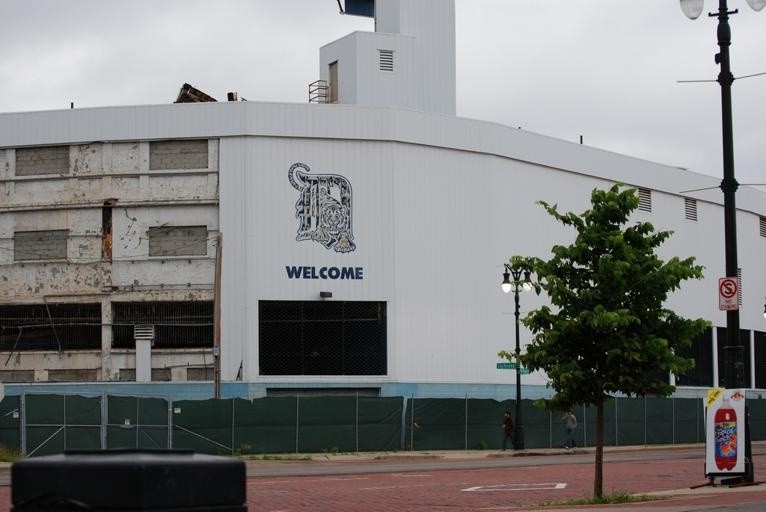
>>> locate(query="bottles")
[714,393,738,470]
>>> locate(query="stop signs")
[718,277,739,311]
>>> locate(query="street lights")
[501,260,533,451]
[676,0,766,490]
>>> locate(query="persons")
[500,411,515,452]
[562,410,577,449]
